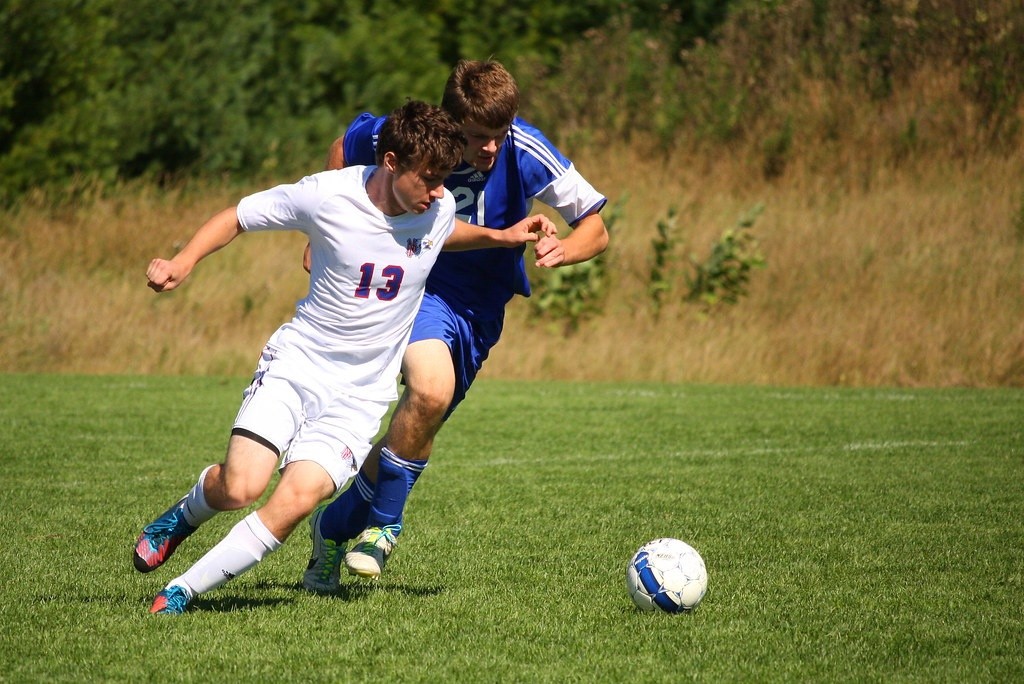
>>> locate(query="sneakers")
[345,525,401,577]
[303,510,349,594]
[133,494,194,573]
[149,586,190,616]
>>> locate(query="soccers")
[627,537,707,615]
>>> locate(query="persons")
[303,54,609,592]
[133,99,557,616]
